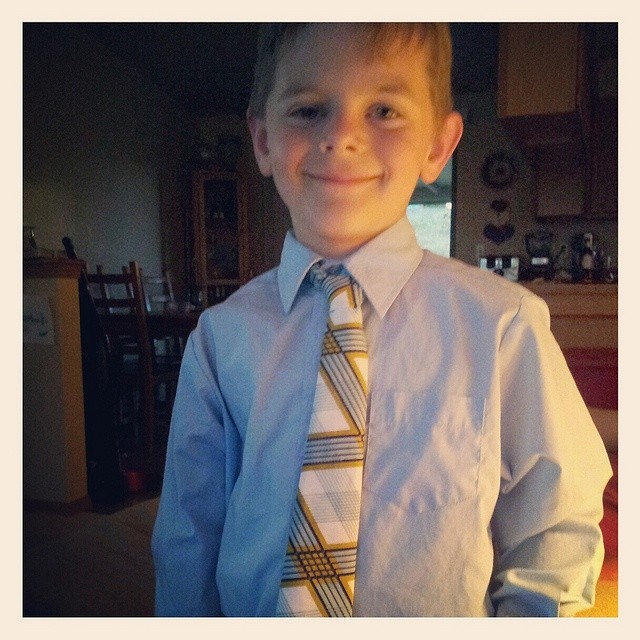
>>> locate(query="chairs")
[95,261,183,477]
[138,267,182,357]
[98,265,146,362]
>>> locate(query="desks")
[110,311,198,329]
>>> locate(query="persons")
[151,21,613,618]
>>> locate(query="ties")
[274,268,366,616]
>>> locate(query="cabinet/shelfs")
[525,280,615,450]
[158,159,250,302]
[497,23,582,116]
